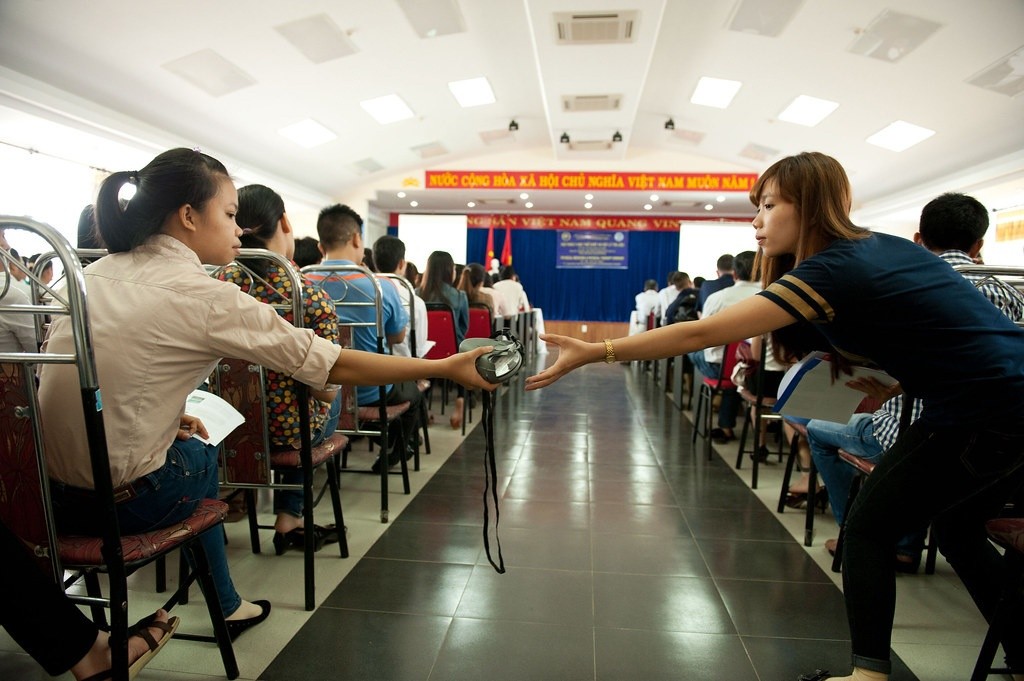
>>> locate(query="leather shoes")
[224,600,271,641]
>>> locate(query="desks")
[529,308,546,353]
[628,311,646,336]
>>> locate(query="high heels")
[786,485,829,515]
[750,446,770,466]
[273,523,348,556]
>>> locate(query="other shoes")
[711,427,737,444]
[826,539,919,574]
[371,437,423,471]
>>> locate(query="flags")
[485,225,494,270]
[500,223,512,267]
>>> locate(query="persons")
[36,147,498,647]
[0,520,180,681]
[524,152,1024,681]
[76,183,530,535]
[620,192,1024,576]
[0,231,53,391]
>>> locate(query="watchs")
[603,339,615,364]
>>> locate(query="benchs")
[645,264,1024,681]
[0,215,536,681]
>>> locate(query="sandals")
[82,609,181,680]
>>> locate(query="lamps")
[509,121,518,130]
[560,132,569,143]
[666,117,674,129]
[613,129,622,140]
